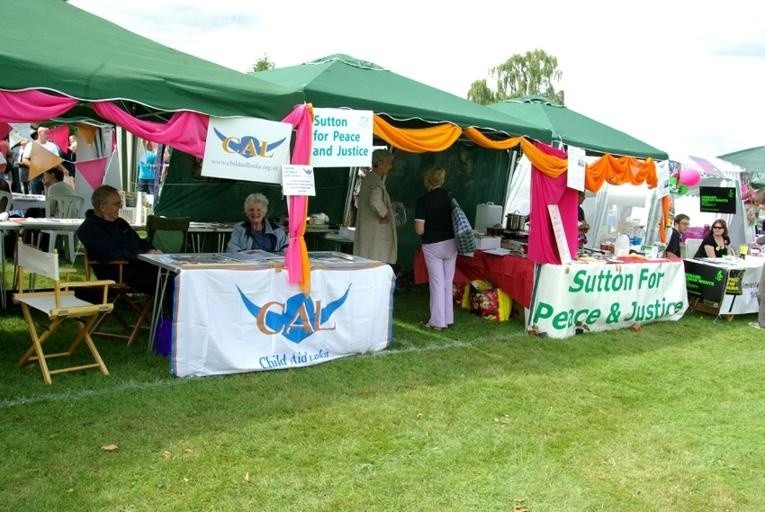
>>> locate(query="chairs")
[30,193,85,264]
[0,191,14,264]
[13,237,116,386]
[83,244,161,347]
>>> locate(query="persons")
[350,166,370,227]
[664,214,690,258]
[413,164,461,330]
[75,184,175,318]
[578,189,590,255]
[353,149,399,273]
[693,219,733,258]
[225,193,290,254]
[749,189,765,329]
[0,126,169,261]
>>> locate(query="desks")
[682,254,764,324]
[0,218,353,308]
[11,192,46,209]
[454,240,686,339]
[136,250,398,378]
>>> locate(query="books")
[155,250,377,268]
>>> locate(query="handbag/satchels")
[451,198,476,253]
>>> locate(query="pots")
[505,208,527,232]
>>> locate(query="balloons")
[679,169,701,189]
[670,173,689,195]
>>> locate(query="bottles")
[613,233,630,258]
[634,226,643,245]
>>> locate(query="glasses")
[713,227,723,229]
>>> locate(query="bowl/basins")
[486,227,503,236]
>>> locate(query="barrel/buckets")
[614,232,630,256]
[474,201,503,233]
